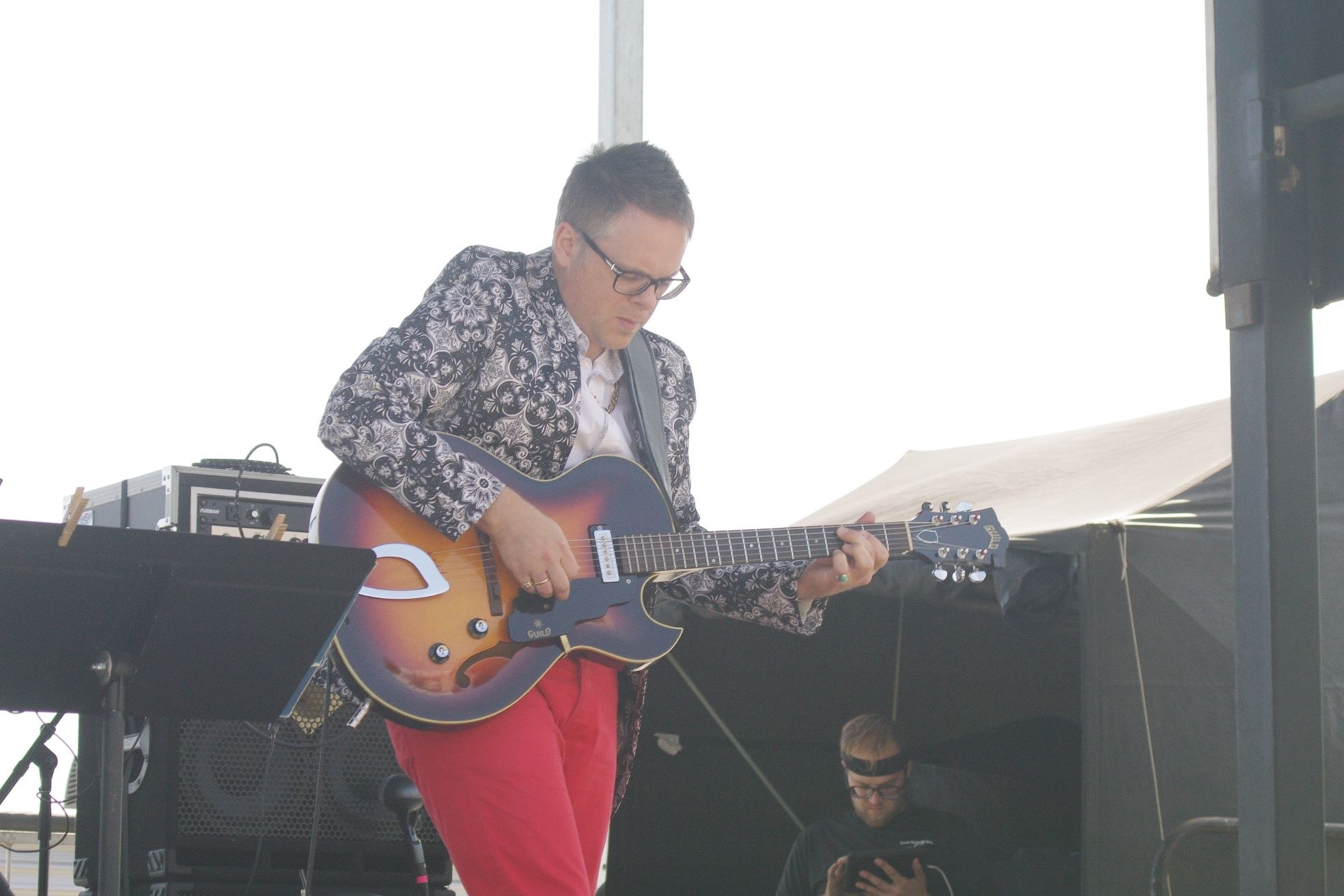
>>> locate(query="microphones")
[375,773,430,887]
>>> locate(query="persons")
[319,139,888,896]
[778,714,992,896]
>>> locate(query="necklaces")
[590,351,620,413]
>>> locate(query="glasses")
[843,767,909,798]
[565,217,690,300]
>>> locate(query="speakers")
[75,652,452,885]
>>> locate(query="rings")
[835,574,853,584]
[535,578,549,586]
[521,579,534,589]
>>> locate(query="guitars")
[309,426,1009,733]
